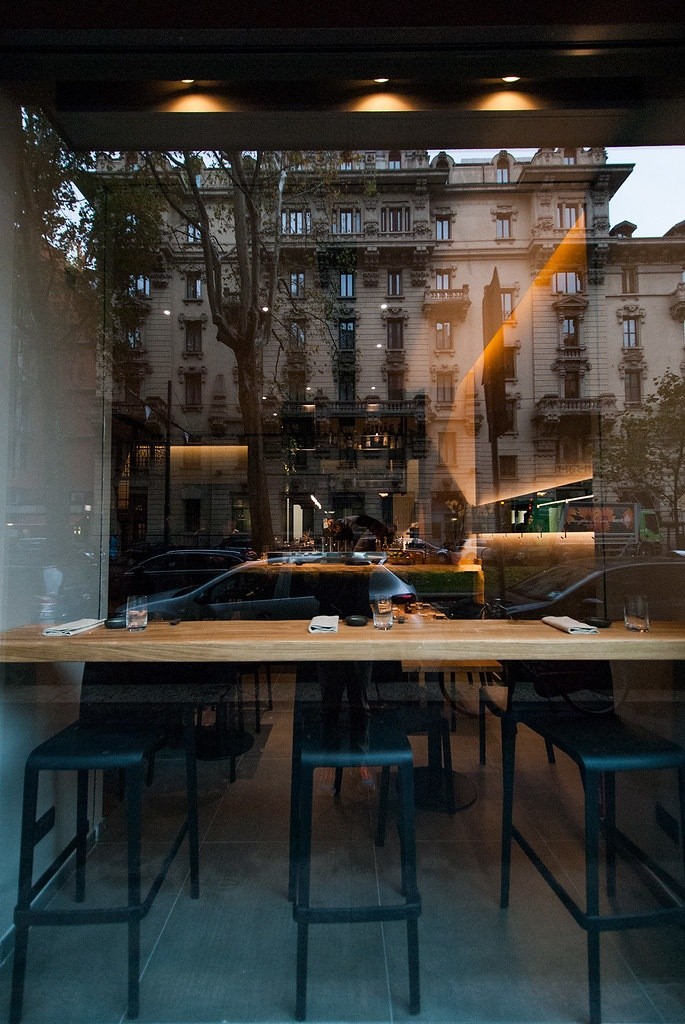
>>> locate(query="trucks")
[531,502,665,557]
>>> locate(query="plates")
[104,618,126,629]
[345,615,368,625]
[584,616,612,629]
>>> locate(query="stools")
[8,662,198,1020]
[499,660,685,1020]
[286,662,424,1023]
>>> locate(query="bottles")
[327,424,402,451]
[269,537,314,552]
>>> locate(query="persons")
[315,566,380,799]
[328,519,354,545]
[220,514,238,541]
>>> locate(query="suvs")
[122,550,251,607]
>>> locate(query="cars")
[445,558,685,651]
[387,536,453,564]
[118,553,423,634]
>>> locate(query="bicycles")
[437,596,630,718]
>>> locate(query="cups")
[372,594,393,630]
[126,595,148,632]
[623,593,651,631]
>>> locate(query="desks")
[1,617,685,1022]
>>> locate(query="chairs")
[136,642,568,814]
